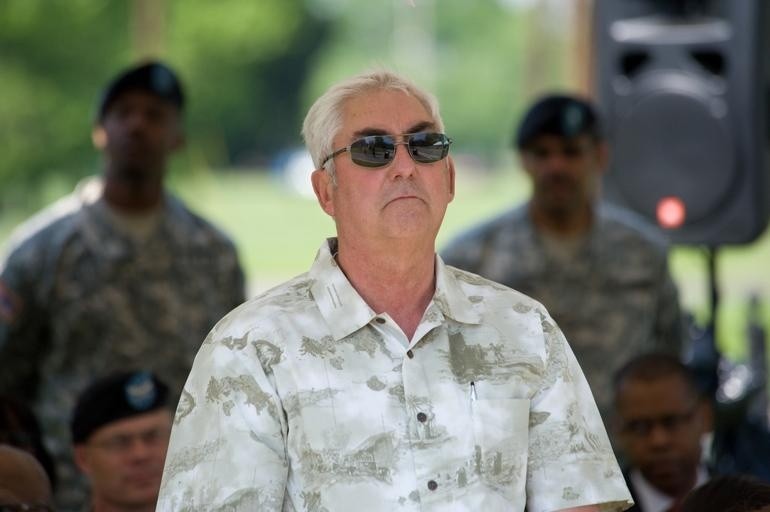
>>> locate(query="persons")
[610,354,737,510]
[0,440,63,511]
[70,367,177,512]
[437,90,724,468]
[672,472,770,512]
[1,57,246,511]
[154,65,636,512]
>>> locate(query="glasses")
[322,132,453,167]
[620,394,703,433]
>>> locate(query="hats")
[517,97,604,149]
[70,368,170,446]
[98,64,185,118]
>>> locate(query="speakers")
[588,0,769,242]
